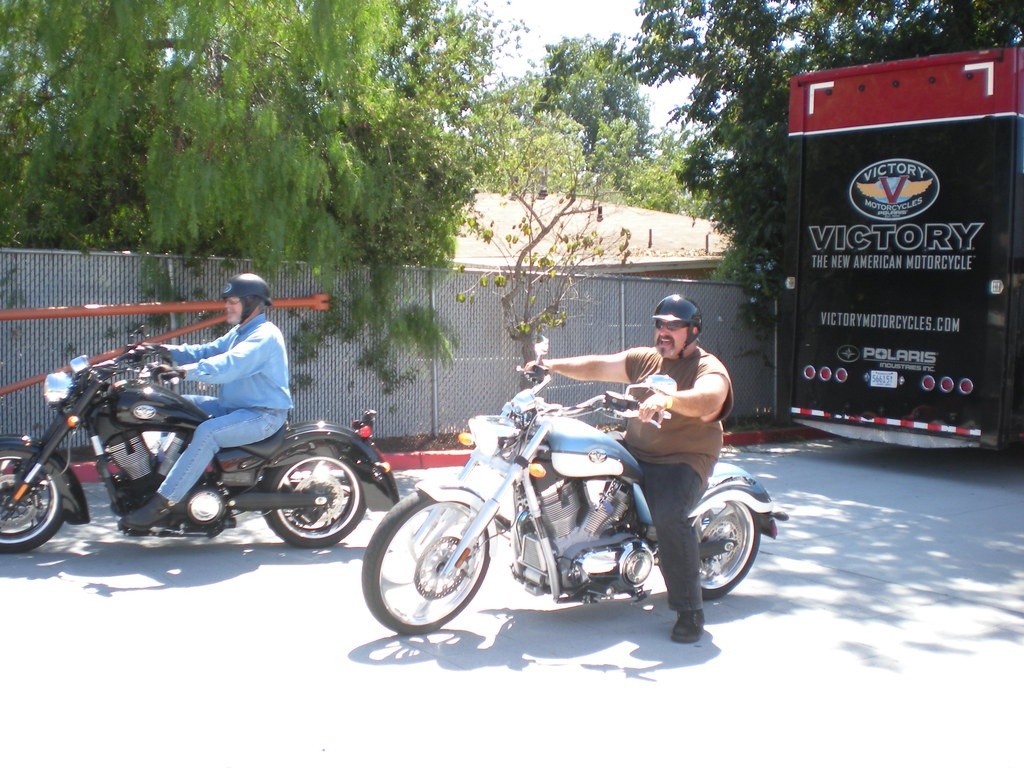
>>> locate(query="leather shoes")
[120,496,172,532]
[671,610,705,643]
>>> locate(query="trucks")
[781,45,1023,455]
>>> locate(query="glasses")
[656,320,689,330]
[225,296,242,305]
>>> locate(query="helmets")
[223,272,272,306]
[651,293,703,333]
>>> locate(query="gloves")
[150,364,186,384]
[135,341,167,358]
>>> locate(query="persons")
[119,273,293,532]
[525,295,734,643]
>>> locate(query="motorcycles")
[361,335,789,637]
[1,324,400,549]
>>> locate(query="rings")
[526,364,529,366]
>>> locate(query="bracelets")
[666,395,672,409]
